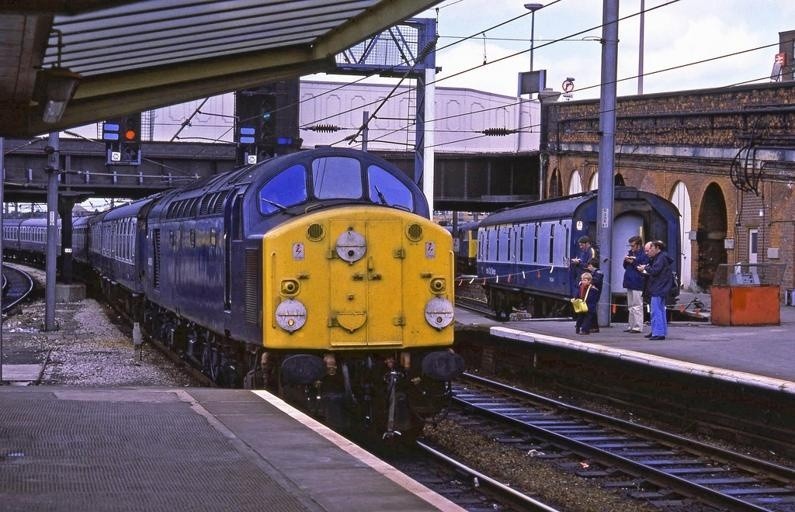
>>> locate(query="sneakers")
[623,329,642,333]
[644,331,665,340]
[573,323,599,336]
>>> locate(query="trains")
[440,185,683,323]
[1,144,464,447]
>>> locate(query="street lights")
[524,4,545,99]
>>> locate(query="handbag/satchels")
[570,298,588,314]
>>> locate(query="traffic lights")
[120,114,142,143]
[260,95,277,129]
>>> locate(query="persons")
[623,236,650,333]
[571,235,604,334]
[637,240,673,341]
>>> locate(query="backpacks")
[670,271,680,297]
